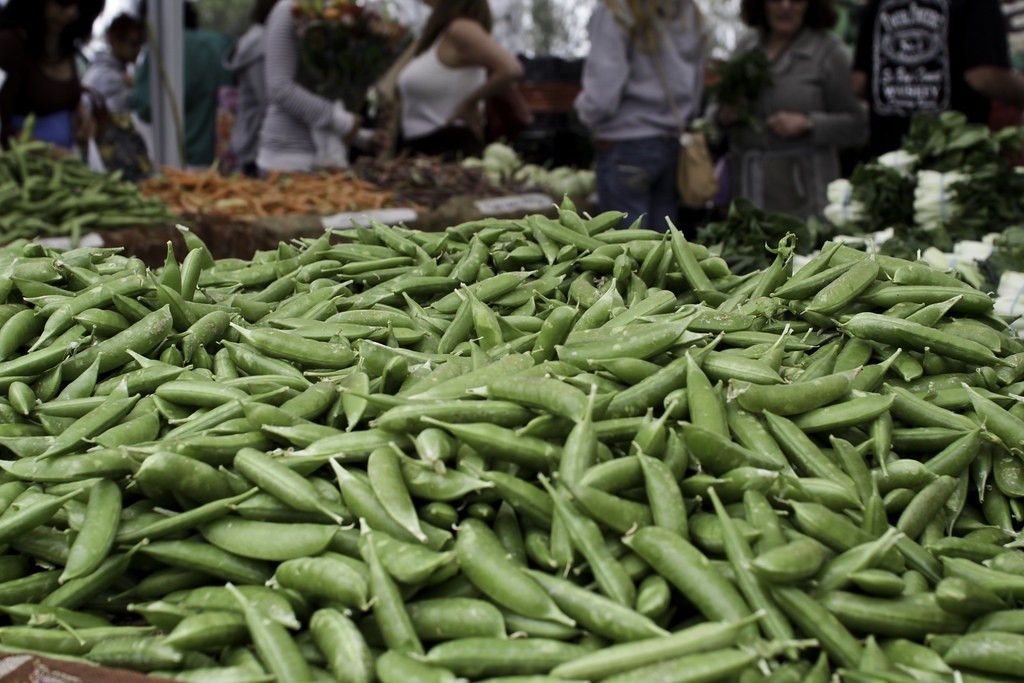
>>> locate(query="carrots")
[141,159,392,217]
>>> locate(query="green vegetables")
[693,107,1024,342]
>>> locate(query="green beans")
[0,193,1024,683]
[0,114,172,248]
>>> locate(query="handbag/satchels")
[488,69,534,137]
[678,133,720,208]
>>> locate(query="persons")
[704,0,869,226]
[81,15,145,173]
[219,0,282,178]
[255,0,391,175]
[0,0,106,150]
[569,0,713,237]
[383,0,524,161]
[119,0,240,167]
[845,0,1024,162]
